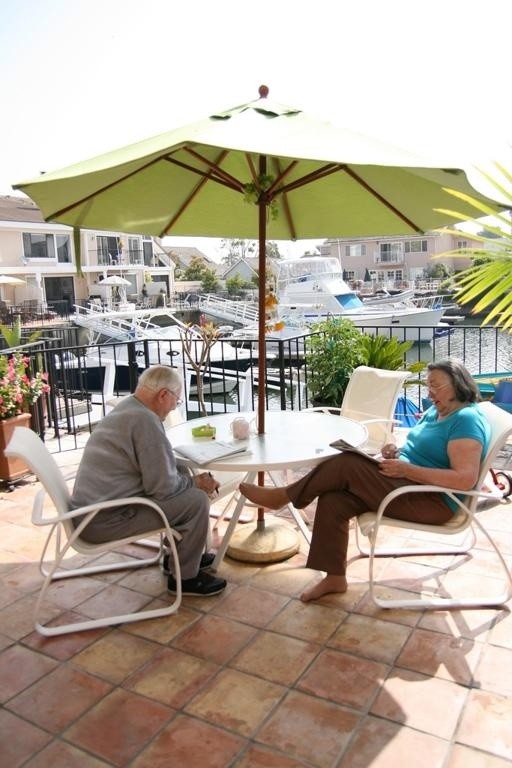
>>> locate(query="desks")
[159,405,372,565]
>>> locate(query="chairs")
[108,251,118,263]
[85,290,153,312]
[355,396,512,611]
[280,363,412,524]
[3,423,188,639]
[108,392,255,555]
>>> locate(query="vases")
[0,411,37,483]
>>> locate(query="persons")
[67,365,227,597]
[238,358,492,602]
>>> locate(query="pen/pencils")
[209,472,219,496]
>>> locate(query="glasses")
[424,381,452,394]
[168,389,184,405]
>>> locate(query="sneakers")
[162,551,217,576]
[167,569,228,597]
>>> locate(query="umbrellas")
[11,86,512,530]
[97,274,132,302]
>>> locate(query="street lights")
[117,240,125,278]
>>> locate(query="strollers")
[413,410,511,498]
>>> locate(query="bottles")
[229,416,249,441]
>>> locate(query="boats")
[54,320,312,396]
[270,257,465,344]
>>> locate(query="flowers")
[0,347,54,420]
